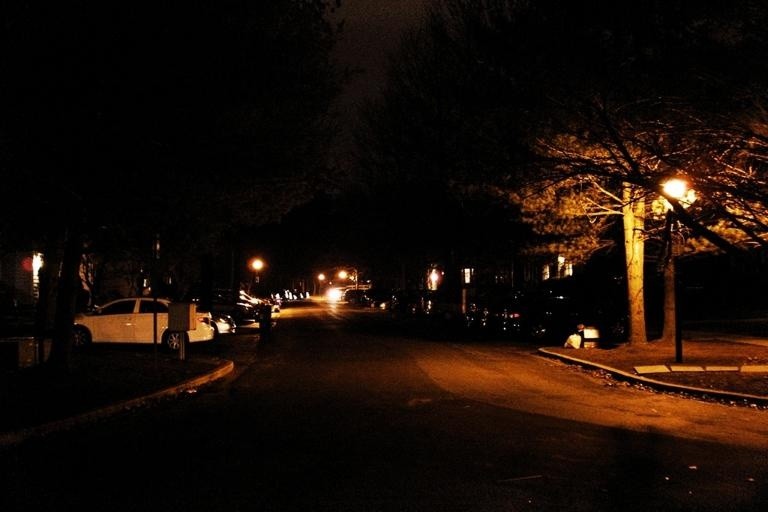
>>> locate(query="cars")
[274,284,309,301]
[213,286,281,322]
[465,284,628,340]
[68,295,213,354]
[213,309,237,336]
[342,288,370,303]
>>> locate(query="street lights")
[657,173,704,363]
